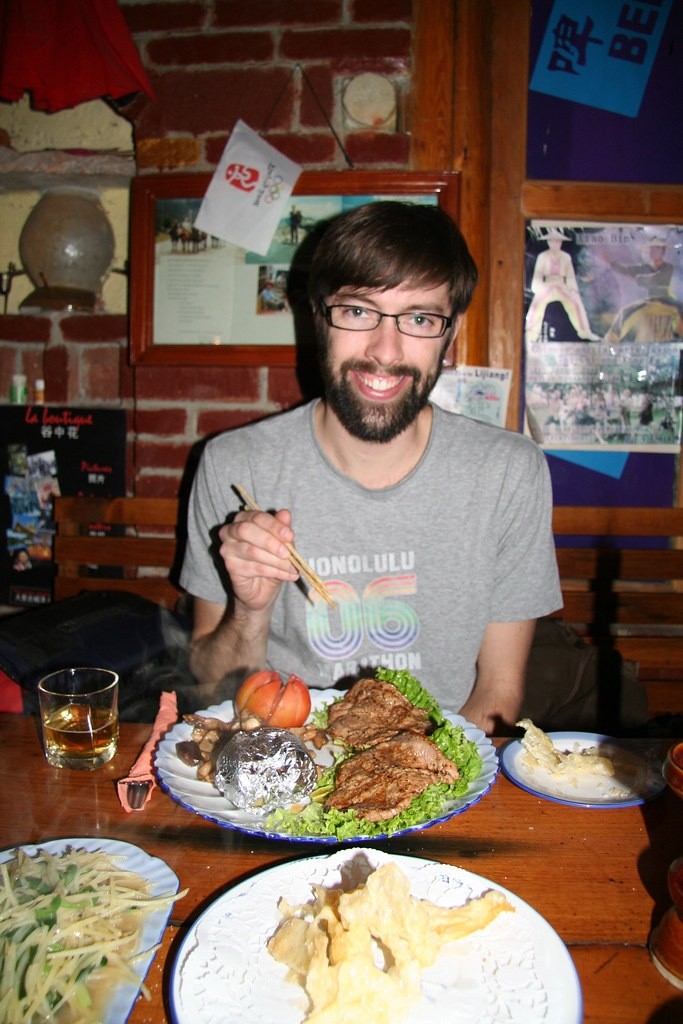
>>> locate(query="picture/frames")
[129,170,465,369]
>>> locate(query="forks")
[126,783,148,810]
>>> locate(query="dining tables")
[0,713,683,1024]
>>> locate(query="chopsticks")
[232,482,335,609]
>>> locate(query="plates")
[152,689,499,844]
[0,838,179,1024]
[166,854,582,1024]
[499,731,667,809]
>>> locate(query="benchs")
[550,507,682,711]
[53,495,185,611]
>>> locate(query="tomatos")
[235,670,312,727]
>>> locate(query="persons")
[276,271,286,288]
[590,238,682,343]
[290,205,302,245]
[523,229,601,342]
[177,201,564,737]
[541,384,679,445]
[182,217,192,233]
[261,282,288,312]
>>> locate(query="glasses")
[318,293,459,339]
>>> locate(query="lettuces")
[267,667,482,839]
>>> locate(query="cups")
[40,667,120,768]
